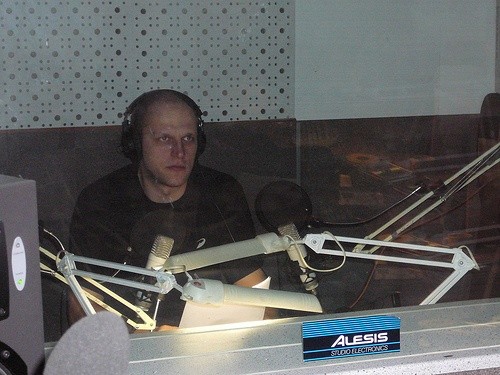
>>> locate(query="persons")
[69,90,278,333]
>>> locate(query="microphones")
[135,233,175,324]
[277,222,319,292]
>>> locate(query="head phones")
[120,89,207,163]
